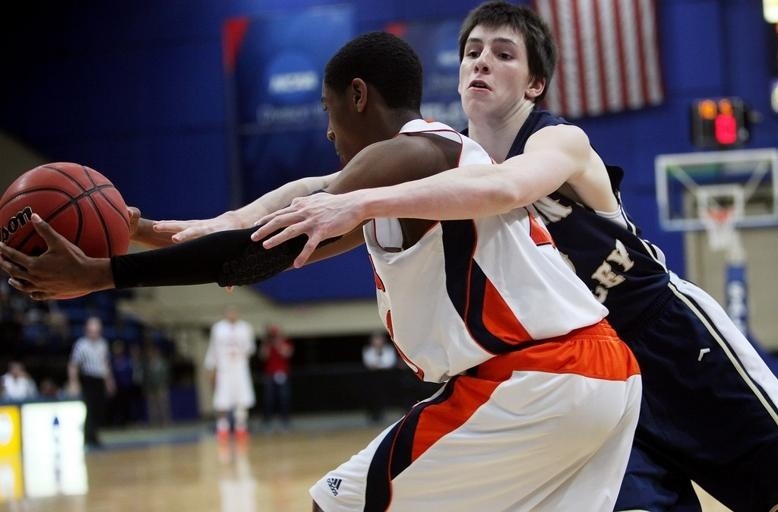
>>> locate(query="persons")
[259,323,293,424]
[202,305,257,456]
[0,274,171,446]
[0,32,646,511]
[147,0,778,511]
[362,332,395,422]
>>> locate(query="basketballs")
[0,161,131,301]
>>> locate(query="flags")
[532,1,664,119]
